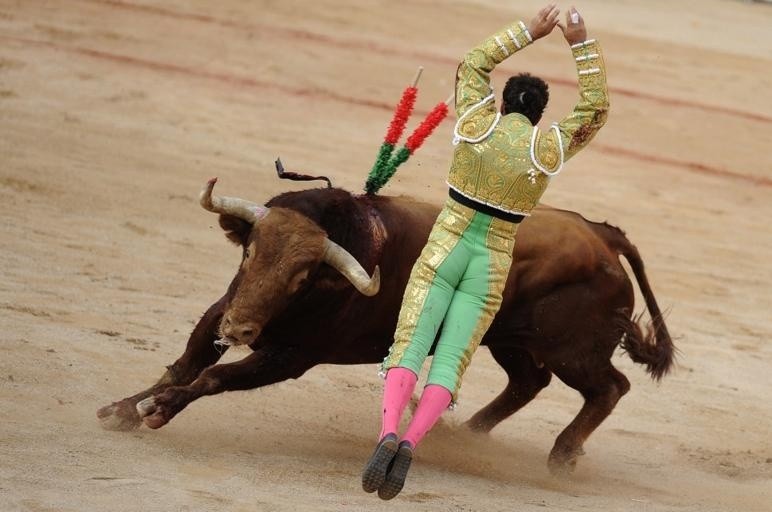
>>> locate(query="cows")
[95,173,688,480]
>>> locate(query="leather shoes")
[362,433,413,500]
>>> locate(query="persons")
[361,2,610,500]
